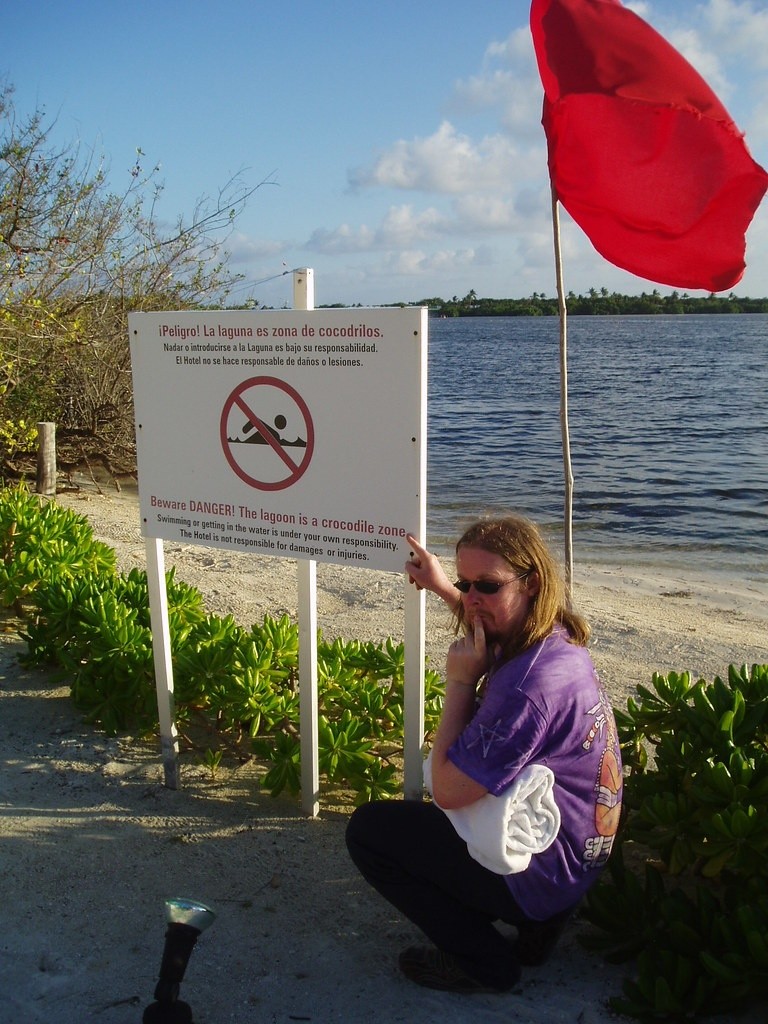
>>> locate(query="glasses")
[453,573,532,594]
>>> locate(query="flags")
[528,0,768,292]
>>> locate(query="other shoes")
[518,923,555,967]
[399,940,522,995]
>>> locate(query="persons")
[346,508,624,993]
